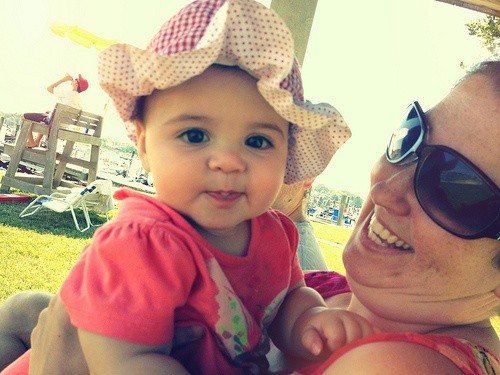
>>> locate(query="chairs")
[19,179,112,233]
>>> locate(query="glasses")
[385,99,500,242]
[75,79,78,83]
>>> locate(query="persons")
[415,148,459,219]
[59,1,380,374]
[0,59,500,375]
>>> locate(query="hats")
[79,74,88,91]
[97,0,352,185]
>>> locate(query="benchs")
[0,103,104,196]
